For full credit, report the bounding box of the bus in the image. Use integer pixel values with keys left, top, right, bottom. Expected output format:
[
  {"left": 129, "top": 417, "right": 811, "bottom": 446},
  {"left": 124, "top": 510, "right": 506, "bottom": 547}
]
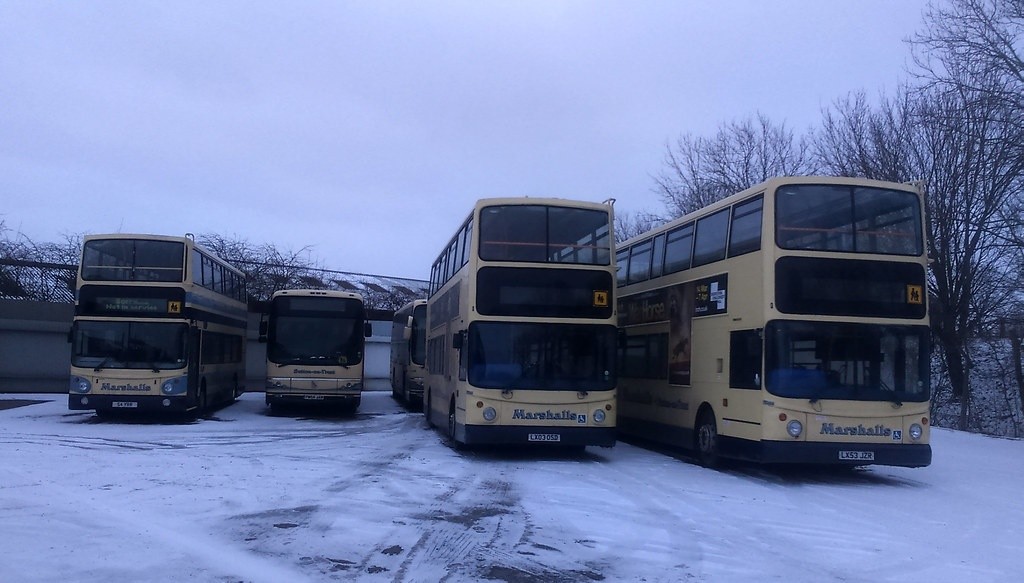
[
  {"left": 390, "top": 299, "right": 426, "bottom": 407},
  {"left": 68, "top": 233, "right": 248, "bottom": 419},
  {"left": 258, "top": 289, "right": 372, "bottom": 407},
  {"left": 425, "top": 197, "right": 621, "bottom": 454},
  {"left": 618, "top": 177, "right": 933, "bottom": 468}
]
